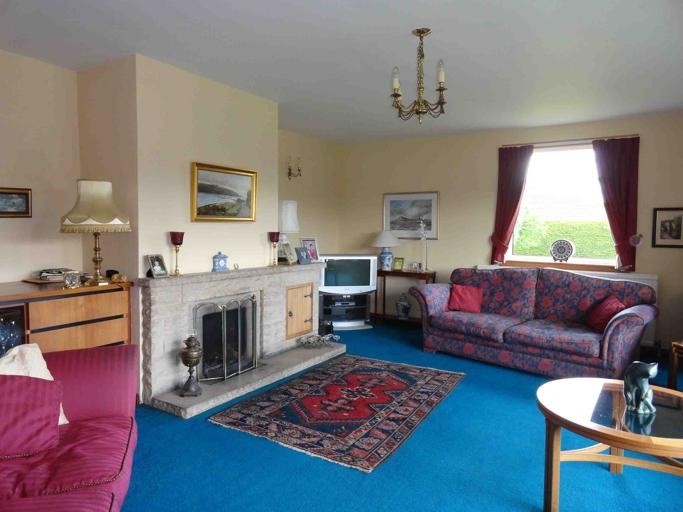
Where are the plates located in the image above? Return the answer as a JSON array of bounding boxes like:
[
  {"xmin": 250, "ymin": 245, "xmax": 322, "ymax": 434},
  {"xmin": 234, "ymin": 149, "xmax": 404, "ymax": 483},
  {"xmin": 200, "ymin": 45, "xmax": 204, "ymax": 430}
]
[{"xmin": 551, "ymin": 239, "xmax": 572, "ymax": 260}]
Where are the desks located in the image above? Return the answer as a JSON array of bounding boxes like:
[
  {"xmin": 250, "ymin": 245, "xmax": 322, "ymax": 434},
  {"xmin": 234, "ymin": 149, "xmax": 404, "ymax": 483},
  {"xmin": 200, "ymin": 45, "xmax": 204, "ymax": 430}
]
[{"xmin": 374, "ymin": 267, "xmax": 436, "ymax": 324}]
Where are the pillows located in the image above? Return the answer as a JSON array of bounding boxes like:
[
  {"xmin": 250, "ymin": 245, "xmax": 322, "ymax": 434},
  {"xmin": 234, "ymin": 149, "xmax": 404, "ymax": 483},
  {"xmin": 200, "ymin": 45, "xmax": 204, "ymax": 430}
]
[
  {"xmin": 448, "ymin": 281, "xmax": 484, "ymax": 313},
  {"xmin": 582, "ymin": 294, "xmax": 625, "ymax": 334},
  {"xmin": 1, "ymin": 345, "xmax": 69, "ymax": 462}
]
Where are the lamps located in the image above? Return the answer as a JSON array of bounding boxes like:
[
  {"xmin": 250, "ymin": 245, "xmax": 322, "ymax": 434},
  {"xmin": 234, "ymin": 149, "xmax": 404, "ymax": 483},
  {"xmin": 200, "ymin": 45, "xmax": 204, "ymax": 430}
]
[
  {"xmin": 169, "ymin": 232, "xmax": 186, "ymax": 278},
  {"xmin": 58, "ymin": 180, "xmax": 133, "ymax": 287},
  {"xmin": 269, "ymin": 232, "xmax": 282, "ymax": 268},
  {"xmin": 368, "ymin": 231, "xmax": 402, "ymax": 271},
  {"xmin": 390, "ymin": 27, "xmax": 448, "ymax": 125}
]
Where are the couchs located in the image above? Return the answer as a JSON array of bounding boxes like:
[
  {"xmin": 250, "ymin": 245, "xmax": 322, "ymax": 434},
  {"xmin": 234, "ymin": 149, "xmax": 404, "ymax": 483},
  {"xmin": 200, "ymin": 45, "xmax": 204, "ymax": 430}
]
[{"xmin": 409, "ymin": 267, "xmax": 661, "ymax": 380}]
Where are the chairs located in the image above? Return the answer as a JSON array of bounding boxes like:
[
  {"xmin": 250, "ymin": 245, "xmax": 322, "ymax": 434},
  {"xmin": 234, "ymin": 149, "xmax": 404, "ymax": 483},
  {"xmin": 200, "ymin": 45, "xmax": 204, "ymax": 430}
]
[{"xmin": 1, "ymin": 344, "xmax": 141, "ymax": 512}]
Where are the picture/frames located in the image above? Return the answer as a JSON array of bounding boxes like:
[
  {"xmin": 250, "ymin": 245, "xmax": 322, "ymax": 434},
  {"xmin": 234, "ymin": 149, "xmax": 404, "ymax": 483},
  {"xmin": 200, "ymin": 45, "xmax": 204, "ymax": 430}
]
[
  {"xmin": 652, "ymin": 207, "xmax": 683, "ymax": 248},
  {"xmin": 383, "ymin": 190, "xmax": 440, "ymax": 241},
  {"xmin": 0, "ymin": 187, "xmax": 33, "ymax": 218},
  {"xmin": 146, "ymin": 253, "xmax": 169, "ymax": 279},
  {"xmin": 282, "ymin": 237, "xmax": 322, "ymax": 266},
  {"xmin": 190, "ymin": 160, "xmax": 258, "ymax": 222}
]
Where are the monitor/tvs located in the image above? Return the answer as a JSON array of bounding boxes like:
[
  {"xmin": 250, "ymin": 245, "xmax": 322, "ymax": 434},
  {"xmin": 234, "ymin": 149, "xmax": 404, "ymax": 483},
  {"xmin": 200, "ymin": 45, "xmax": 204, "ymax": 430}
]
[{"xmin": 318, "ymin": 256, "xmax": 377, "ymax": 298}]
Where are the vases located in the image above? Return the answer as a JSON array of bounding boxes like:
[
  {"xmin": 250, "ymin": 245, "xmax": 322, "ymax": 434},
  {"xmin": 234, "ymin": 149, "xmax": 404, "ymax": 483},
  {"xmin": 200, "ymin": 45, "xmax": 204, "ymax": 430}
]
[{"xmin": 395, "ymin": 294, "xmax": 411, "ymax": 321}]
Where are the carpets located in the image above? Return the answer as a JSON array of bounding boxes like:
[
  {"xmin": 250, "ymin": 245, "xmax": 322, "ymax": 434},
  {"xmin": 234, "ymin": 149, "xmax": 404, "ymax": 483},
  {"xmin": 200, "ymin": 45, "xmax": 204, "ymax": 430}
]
[{"xmin": 206, "ymin": 351, "xmax": 468, "ymax": 475}]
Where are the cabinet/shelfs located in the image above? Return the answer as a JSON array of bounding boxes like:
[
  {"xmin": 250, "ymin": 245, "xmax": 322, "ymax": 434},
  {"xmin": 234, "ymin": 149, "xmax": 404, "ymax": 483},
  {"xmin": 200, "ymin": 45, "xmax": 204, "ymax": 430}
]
[
  {"xmin": 0, "ymin": 271, "xmax": 134, "ymax": 352},
  {"xmin": 318, "ymin": 290, "xmax": 376, "ymax": 328}
]
